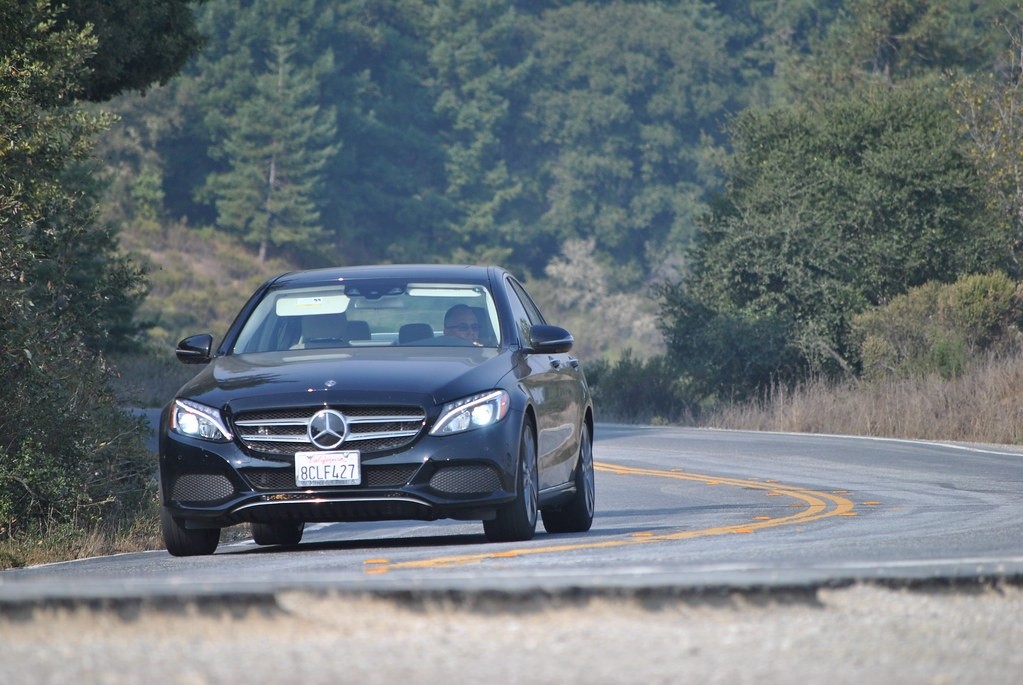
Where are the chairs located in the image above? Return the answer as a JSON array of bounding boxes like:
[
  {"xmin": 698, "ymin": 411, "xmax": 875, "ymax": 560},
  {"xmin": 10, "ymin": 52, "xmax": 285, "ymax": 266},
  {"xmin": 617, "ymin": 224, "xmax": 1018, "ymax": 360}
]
[
  {"xmin": 398, "ymin": 323, "xmax": 434, "ymax": 343},
  {"xmin": 472, "ymin": 307, "xmax": 497, "ymax": 345},
  {"xmin": 347, "ymin": 321, "xmax": 371, "ymax": 340},
  {"xmin": 301, "ymin": 312, "xmax": 352, "ymax": 347}
]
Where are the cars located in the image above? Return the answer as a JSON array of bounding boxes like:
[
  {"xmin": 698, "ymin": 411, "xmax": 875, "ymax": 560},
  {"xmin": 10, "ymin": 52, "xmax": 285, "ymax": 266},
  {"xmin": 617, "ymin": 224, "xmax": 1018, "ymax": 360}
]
[{"xmin": 155, "ymin": 262, "xmax": 595, "ymax": 558}]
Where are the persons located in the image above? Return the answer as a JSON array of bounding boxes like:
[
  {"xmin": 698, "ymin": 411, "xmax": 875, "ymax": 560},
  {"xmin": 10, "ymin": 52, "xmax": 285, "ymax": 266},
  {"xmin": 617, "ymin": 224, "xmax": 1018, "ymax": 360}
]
[{"xmin": 444, "ymin": 304, "xmax": 479, "ymax": 344}]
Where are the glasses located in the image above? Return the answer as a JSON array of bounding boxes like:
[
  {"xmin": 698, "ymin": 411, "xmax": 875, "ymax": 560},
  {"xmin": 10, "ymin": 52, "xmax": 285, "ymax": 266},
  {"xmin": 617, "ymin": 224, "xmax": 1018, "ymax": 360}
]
[{"xmin": 446, "ymin": 323, "xmax": 480, "ymax": 332}]
